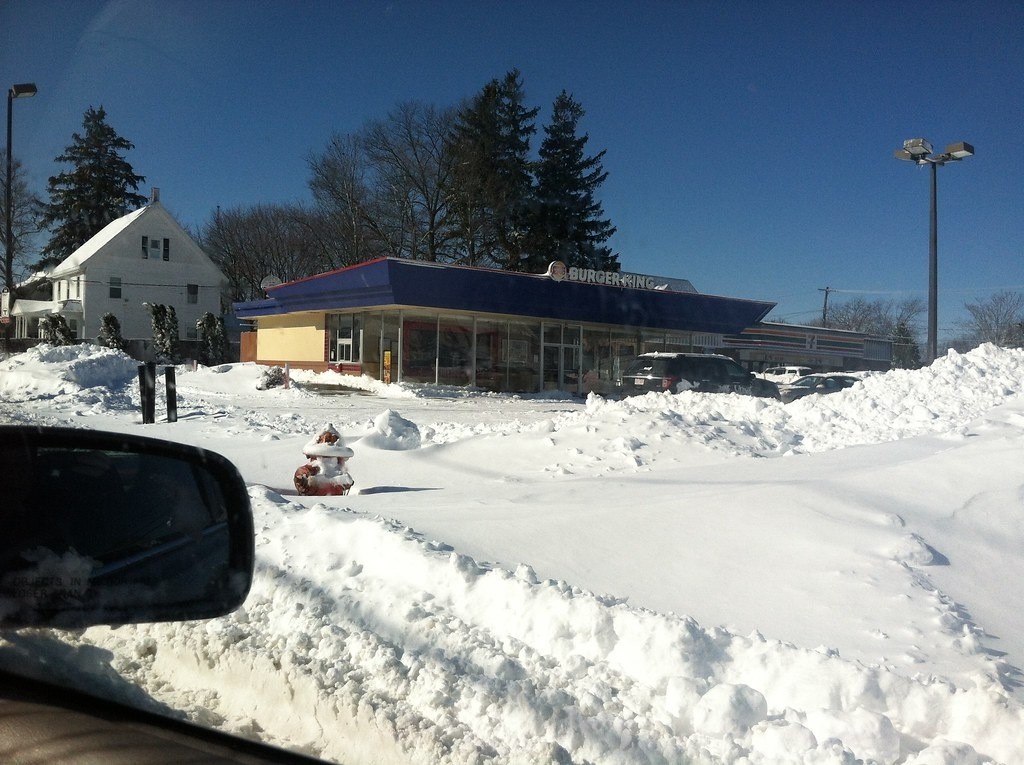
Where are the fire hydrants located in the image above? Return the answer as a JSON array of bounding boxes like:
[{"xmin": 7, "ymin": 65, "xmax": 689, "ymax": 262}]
[{"xmin": 293, "ymin": 424, "xmax": 355, "ymax": 497}]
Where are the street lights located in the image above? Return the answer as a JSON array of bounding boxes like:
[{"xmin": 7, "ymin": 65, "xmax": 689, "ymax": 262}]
[
  {"xmin": 892, "ymin": 138, "xmax": 975, "ymax": 367},
  {"xmin": 6, "ymin": 83, "xmax": 38, "ymax": 290}
]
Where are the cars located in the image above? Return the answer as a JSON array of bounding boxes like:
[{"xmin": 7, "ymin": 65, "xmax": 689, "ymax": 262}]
[
  {"xmin": 780, "ymin": 374, "xmax": 863, "ymax": 405},
  {"xmin": 760, "ymin": 367, "xmax": 813, "ymax": 386},
  {"xmin": 612, "ymin": 350, "xmax": 781, "ymax": 402}
]
